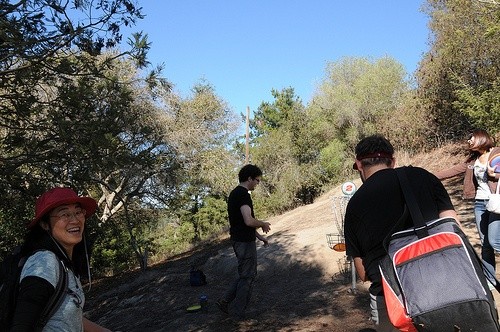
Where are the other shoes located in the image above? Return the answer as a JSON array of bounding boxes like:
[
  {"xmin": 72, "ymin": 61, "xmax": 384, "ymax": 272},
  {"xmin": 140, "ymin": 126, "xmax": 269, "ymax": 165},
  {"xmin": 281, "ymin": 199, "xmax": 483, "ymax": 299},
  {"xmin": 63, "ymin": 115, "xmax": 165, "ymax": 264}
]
[
  {"xmin": 232, "ymin": 319, "xmax": 258, "ymax": 326},
  {"xmin": 215, "ymin": 297, "xmax": 229, "ymax": 313}
]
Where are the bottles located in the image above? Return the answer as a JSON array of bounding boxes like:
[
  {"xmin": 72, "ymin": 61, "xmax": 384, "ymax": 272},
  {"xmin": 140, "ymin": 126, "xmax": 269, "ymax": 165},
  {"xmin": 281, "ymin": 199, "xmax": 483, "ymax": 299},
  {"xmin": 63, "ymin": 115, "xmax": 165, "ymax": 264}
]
[{"xmin": 200, "ymin": 296, "xmax": 208, "ymax": 314}]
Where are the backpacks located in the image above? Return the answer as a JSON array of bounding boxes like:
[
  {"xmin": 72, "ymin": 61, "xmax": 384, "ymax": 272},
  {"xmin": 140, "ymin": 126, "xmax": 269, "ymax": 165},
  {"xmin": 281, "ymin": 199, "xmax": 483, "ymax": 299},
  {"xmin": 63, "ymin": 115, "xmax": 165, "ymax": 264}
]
[{"xmin": 0, "ymin": 252, "xmax": 70, "ymax": 332}]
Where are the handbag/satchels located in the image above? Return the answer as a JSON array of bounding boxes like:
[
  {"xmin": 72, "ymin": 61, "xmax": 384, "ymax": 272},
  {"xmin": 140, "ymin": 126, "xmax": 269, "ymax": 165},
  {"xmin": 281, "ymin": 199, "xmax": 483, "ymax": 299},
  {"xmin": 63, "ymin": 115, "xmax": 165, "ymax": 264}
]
[
  {"xmin": 485, "ymin": 194, "xmax": 499, "ymax": 214},
  {"xmin": 378, "ymin": 217, "xmax": 500, "ymax": 332},
  {"xmin": 189, "ymin": 262, "xmax": 207, "ymax": 286}
]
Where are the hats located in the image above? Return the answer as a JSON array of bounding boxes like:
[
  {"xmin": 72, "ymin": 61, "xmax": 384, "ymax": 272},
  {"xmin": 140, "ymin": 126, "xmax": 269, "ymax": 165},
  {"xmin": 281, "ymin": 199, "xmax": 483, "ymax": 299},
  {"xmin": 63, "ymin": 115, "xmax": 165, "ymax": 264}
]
[
  {"xmin": 353, "ymin": 152, "xmax": 393, "ymax": 170},
  {"xmin": 24, "ymin": 187, "xmax": 97, "ymax": 231}
]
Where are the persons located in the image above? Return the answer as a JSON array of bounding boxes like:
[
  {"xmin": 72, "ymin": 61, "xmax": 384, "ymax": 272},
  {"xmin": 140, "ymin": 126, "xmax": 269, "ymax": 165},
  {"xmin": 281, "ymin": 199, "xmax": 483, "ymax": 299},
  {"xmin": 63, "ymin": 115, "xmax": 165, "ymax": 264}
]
[
  {"xmin": 343, "ymin": 133, "xmax": 464, "ymax": 332},
  {"xmin": 433, "ymin": 128, "xmax": 500, "ymax": 291},
  {"xmin": 11, "ymin": 187, "xmax": 111, "ymax": 332},
  {"xmin": 215, "ymin": 164, "xmax": 271, "ymax": 326}
]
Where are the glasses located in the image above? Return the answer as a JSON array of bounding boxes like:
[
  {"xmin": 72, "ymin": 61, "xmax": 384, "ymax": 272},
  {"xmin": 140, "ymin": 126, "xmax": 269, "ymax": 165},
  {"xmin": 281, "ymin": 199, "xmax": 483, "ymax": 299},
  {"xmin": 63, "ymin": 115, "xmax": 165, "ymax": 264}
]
[
  {"xmin": 254, "ymin": 177, "xmax": 261, "ymax": 183},
  {"xmin": 48, "ymin": 209, "xmax": 87, "ymax": 220}
]
